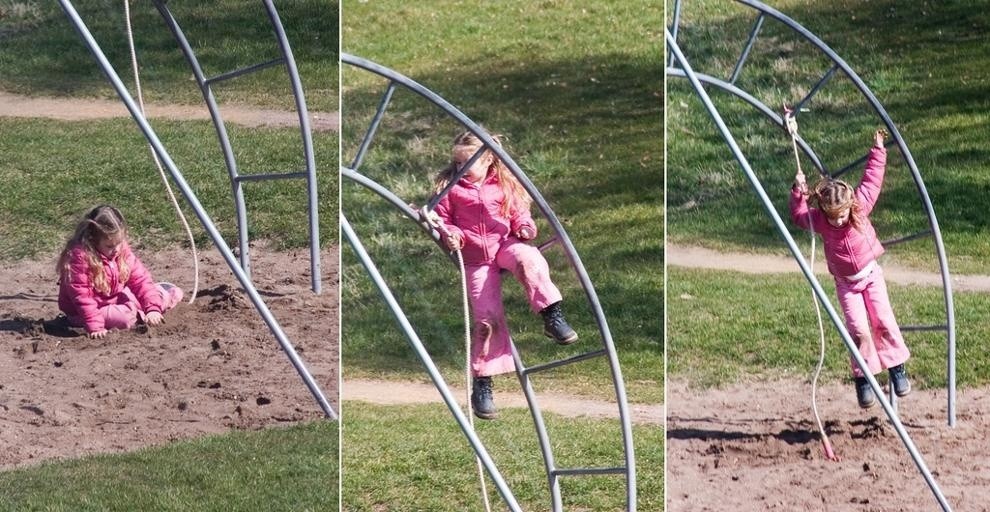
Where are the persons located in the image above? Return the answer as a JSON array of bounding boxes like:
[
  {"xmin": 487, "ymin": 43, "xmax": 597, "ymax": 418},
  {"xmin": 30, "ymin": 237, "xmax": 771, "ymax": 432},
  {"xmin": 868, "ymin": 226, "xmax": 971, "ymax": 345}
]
[
  {"xmin": 53, "ymin": 202, "xmax": 186, "ymax": 343},
  {"xmin": 425, "ymin": 128, "xmax": 579, "ymax": 423},
  {"xmin": 786, "ymin": 123, "xmax": 913, "ymax": 409}
]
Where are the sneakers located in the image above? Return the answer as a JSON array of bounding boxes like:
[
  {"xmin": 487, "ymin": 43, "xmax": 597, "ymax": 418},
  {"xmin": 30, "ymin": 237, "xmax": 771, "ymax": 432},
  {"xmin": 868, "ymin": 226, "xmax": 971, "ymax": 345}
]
[
  {"xmin": 853, "ymin": 376, "xmax": 876, "ymax": 408},
  {"xmin": 887, "ymin": 362, "xmax": 911, "ymax": 396},
  {"xmin": 540, "ymin": 302, "xmax": 579, "ymax": 345},
  {"xmin": 471, "ymin": 375, "xmax": 498, "ymax": 420}
]
